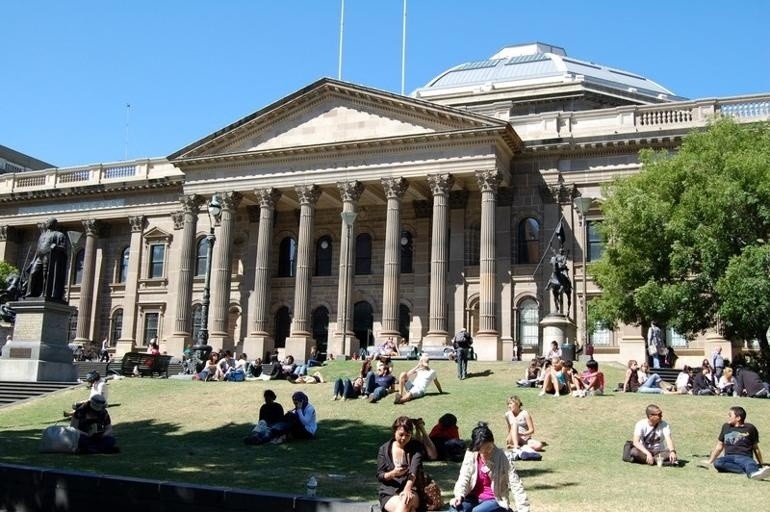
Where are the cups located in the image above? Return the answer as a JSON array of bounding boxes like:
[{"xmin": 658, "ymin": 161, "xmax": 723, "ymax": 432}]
[{"xmin": 656, "ymin": 457, "xmax": 664, "ymax": 469}]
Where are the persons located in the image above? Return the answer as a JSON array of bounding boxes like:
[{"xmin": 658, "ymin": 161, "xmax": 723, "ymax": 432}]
[
  {"xmin": 413, "ymin": 418, "xmax": 466, "ymax": 463},
  {"xmin": 550, "ymin": 247, "xmax": 571, "ymax": 289},
  {"xmin": 452, "ymin": 328, "xmax": 469, "ymax": 380},
  {"xmin": 327, "ymin": 336, "xmax": 441, "ymax": 402},
  {"xmin": 100, "ymin": 335, "xmax": 110, "ymax": 362},
  {"xmin": 429, "ymin": 412, "xmax": 460, "ymax": 440},
  {"xmin": 259, "ymin": 388, "xmax": 284, "ymax": 426},
  {"xmin": 5, "ymin": 334, "xmax": 12, "ymax": 344},
  {"xmin": 70, "ymin": 394, "xmax": 121, "ymax": 454},
  {"xmin": 24, "ymin": 216, "xmax": 67, "ymax": 297},
  {"xmin": 376, "ymin": 415, "xmax": 426, "ymax": 512},
  {"xmin": 447, "ymin": 420, "xmax": 530, "ymax": 512},
  {"xmin": 515, "ymin": 341, "xmax": 604, "ymax": 397},
  {"xmin": 63, "ymin": 370, "xmax": 108, "ymax": 417},
  {"xmin": 131, "ymin": 334, "xmax": 159, "ymax": 377},
  {"xmin": 270, "ymin": 390, "xmax": 318, "ymax": 445},
  {"xmin": 620, "ymin": 346, "xmax": 770, "ymax": 399},
  {"xmin": 704, "ymin": 407, "xmax": 770, "ymax": 481},
  {"xmin": 630, "ymin": 403, "xmax": 678, "ymax": 466},
  {"xmin": 504, "ymin": 395, "xmax": 534, "ymax": 449},
  {"xmin": 648, "ymin": 320, "xmax": 665, "ymax": 368},
  {"xmin": 184, "ymin": 344, "xmax": 324, "ymax": 385}
]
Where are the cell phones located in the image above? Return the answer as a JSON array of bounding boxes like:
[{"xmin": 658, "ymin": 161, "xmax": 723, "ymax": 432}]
[{"xmin": 401, "ymin": 464, "xmax": 408, "ymax": 471}]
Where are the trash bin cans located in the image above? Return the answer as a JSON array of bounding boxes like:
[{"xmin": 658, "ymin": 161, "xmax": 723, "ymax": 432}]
[{"xmin": 561, "ymin": 343, "xmax": 576, "ymax": 361}]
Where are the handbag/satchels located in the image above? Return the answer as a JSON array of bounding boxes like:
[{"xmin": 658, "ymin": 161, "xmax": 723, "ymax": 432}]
[
  {"xmin": 623, "ymin": 441, "xmax": 634, "ymax": 462},
  {"xmin": 39, "ymin": 423, "xmax": 81, "ymax": 453},
  {"xmin": 424, "ymin": 479, "xmax": 442, "ymax": 511},
  {"xmin": 647, "ymin": 345, "xmax": 657, "ymax": 356}
]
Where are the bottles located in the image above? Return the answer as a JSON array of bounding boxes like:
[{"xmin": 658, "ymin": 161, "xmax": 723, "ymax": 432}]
[{"xmin": 306, "ymin": 475, "xmax": 317, "ymax": 497}]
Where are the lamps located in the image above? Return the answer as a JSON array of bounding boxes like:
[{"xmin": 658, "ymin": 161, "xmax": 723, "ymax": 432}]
[{"xmin": 123, "ymin": 251, "xmax": 130, "ymax": 259}]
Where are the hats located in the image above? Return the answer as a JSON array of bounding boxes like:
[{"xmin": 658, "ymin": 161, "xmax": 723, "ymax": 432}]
[{"xmin": 91, "ymin": 394, "xmax": 106, "ymax": 406}]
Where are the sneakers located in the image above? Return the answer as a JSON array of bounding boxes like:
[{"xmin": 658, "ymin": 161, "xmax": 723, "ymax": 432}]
[{"xmin": 751, "ymin": 466, "xmax": 770, "ymax": 480}]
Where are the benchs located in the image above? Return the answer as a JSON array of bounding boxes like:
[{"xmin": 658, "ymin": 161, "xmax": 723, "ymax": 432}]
[
  {"xmin": 109, "ymin": 353, "xmax": 174, "ymax": 379},
  {"xmin": 365, "ymin": 346, "xmax": 418, "ymax": 361},
  {"xmin": 421, "ymin": 346, "xmax": 453, "ymax": 361}
]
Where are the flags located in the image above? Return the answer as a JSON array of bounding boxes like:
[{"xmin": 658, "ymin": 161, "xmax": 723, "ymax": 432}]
[{"xmin": 555, "ymin": 223, "xmax": 566, "ymax": 243}]
